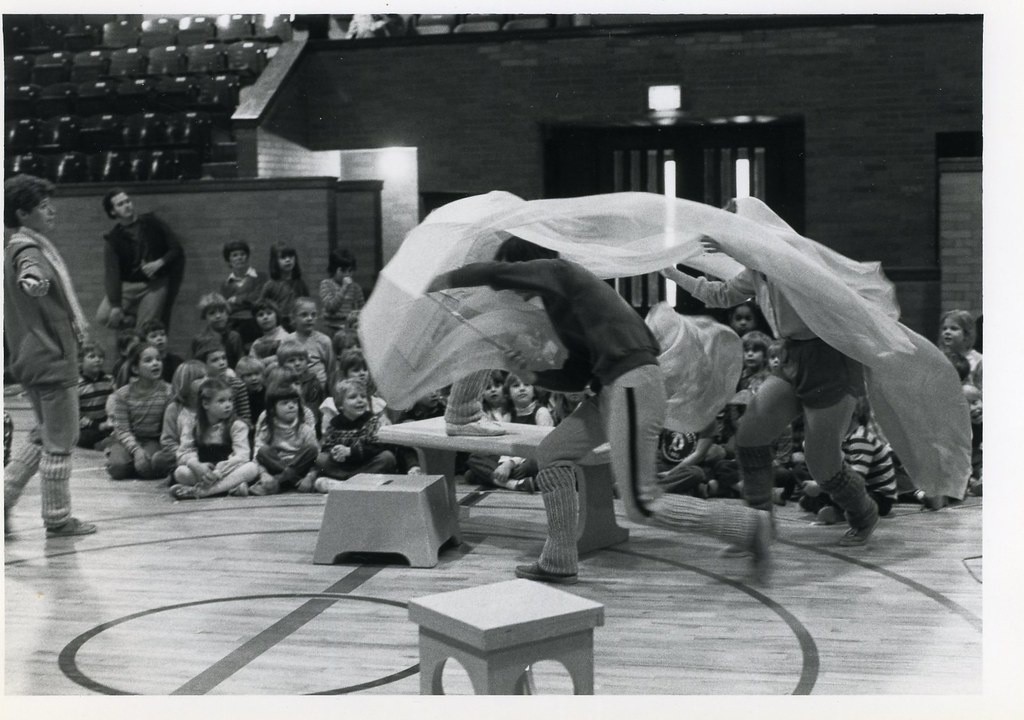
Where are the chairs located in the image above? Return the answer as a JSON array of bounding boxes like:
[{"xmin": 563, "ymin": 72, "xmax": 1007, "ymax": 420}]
[{"xmin": 3, "ymin": 14, "xmax": 554, "ymax": 185}]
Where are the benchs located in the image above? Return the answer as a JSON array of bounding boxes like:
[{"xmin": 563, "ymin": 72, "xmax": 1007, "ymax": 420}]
[{"xmin": 377, "ymin": 415, "xmax": 630, "ymax": 556}]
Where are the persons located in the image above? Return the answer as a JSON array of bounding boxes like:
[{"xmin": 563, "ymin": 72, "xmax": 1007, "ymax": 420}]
[
  {"xmin": 96, "ymin": 187, "xmax": 185, "ymax": 330},
  {"xmin": 220, "ymin": 239, "xmax": 268, "ymax": 343},
  {"xmin": 192, "ymin": 293, "xmax": 241, "ymax": 369},
  {"xmin": 249, "ymin": 302, "xmax": 289, "ymax": 374},
  {"xmin": 316, "ymin": 376, "xmax": 383, "ymax": 481},
  {"xmin": 4, "ymin": 172, "xmax": 97, "ymax": 536},
  {"xmin": 612, "ymin": 303, "xmax": 983, "ymax": 523},
  {"xmin": 268, "ymin": 337, "xmax": 323, "ymax": 422},
  {"xmin": 249, "ymin": 381, "xmax": 320, "ymax": 494},
  {"xmin": 660, "ymin": 197, "xmax": 881, "ymax": 547},
  {"xmin": 319, "ymin": 249, "xmax": 393, "ymax": 433},
  {"xmin": 426, "ymin": 236, "xmax": 772, "ymax": 584},
  {"xmin": 79, "ymin": 343, "xmax": 117, "ymax": 449},
  {"xmin": 236, "ymin": 356, "xmax": 266, "ymax": 423},
  {"xmin": 106, "ymin": 320, "xmax": 265, "ymax": 499},
  {"xmin": 390, "ymin": 367, "xmax": 588, "ymax": 494},
  {"xmin": 289, "ymin": 296, "xmax": 335, "ymax": 398},
  {"xmin": 263, "ymin": 239, "xmax": 310, "ymax": 334}
]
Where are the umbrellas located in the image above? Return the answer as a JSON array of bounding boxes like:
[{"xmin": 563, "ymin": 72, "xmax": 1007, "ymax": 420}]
[{"xmin": 358, "ymin": 190, "xmax": 527, "ymax": 390}]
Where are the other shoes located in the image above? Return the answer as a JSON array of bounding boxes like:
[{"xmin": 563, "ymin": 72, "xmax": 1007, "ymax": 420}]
[
  {"xmin": 970, "ymin": 482, "xmax": 982, "ymax": 496},
  {"xmin": 836, "ymin": 515, "xmax": 882, "ymax": 544},
  {"xmin": 315, "ymin": 477, "xmax": 339, "ymax": 492},
  {"xmin": 445, "ymin": 415, "xmax": 507, "ymax": 436},
  {"xmin": 229, "ymin": 481, "xmax": 248, "ymax": 496},
  {"xmin": 799, "ymin": 496, "xmax": 808, "ymax": 508},
  {"xmin": 45, "ymin": 518, "xmax": 96, "ymax": 536},
  {"xmin": 772, "ymin": 487, "xmax": 786, "ymax": 506},
  {"xmin": 515, "ymin": 561, "xmax": 578, "ymax": 584},
  {"xmin": 696, "ymin": 483, "xmax": 709, "ymax": 498},
  {"xmin": 250, "ymin": 477, "xmax": 282, "ymax": 496},
  {"xmin": 517, "ymin": 476, "xmax": 534, "ymax": 493},
  {"xmin": 818, "ymin": 506, "xmax": 841, "ymax": 523},
  {"xmin": 923, "ymin": 492, "xmax": 950, "ymax": 508},
  {"xmin": 464, "ymin": 469, "xmax": 482, "ymax": 484},
  {"xmin": 169, "ymin": 484, "xmax": 200, "ymax": 499}
]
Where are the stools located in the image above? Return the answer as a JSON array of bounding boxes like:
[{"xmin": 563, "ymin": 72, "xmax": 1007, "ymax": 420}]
[
  {"xmin": 408, "ymin": 578, "xmax": 604, "ymax": 695},
  {"xmin": 313, "ymin": 473, "xmax": 462, "ymax": 568}
]
